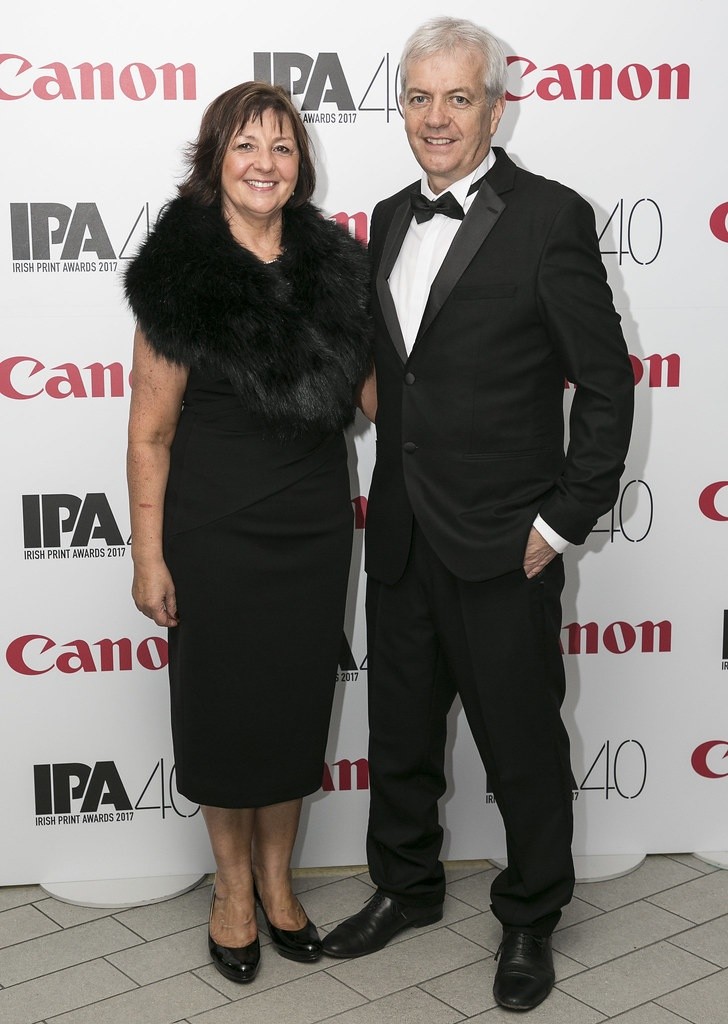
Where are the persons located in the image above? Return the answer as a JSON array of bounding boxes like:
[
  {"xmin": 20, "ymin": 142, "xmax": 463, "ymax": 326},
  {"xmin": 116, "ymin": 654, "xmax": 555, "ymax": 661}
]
[
  {"xmin": 321, "ymin": 18, "xmax": 635, "ymax": 1013},
  {"xmin": 127, "ymin": 83, "xmax": 378, "ymax": 988}
]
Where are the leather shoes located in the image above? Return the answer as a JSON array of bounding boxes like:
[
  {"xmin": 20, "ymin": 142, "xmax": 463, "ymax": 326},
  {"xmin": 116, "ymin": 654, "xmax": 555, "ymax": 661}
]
[
  {"xmin": 493, "ymin": 925, "xmax": 555, "ymax": 1010},
  {"xmin": 321, "ymin": 892, "xmax": 443, "ymax": 958},
  {"xmin": 254, "ymin": 877, "xmax": 323, "ymax": 962},
  {"xmin": 207, "ymin": 879, "xmax": 261, "ymax": 983}
]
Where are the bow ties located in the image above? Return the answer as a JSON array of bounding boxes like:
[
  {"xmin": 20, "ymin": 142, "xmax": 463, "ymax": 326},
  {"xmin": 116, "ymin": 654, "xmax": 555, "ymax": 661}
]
[{"xmin": 407, "ymin": 169, "xmax": 489, "ymax": 225}]
